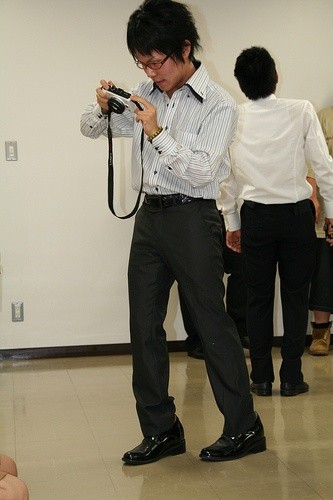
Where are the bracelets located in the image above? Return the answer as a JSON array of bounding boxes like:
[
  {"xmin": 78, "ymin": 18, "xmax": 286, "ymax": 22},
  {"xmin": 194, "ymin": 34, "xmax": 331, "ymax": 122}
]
[{"xmin": 147, "ymin": 127, "xmax": 163, "ymax": 141}]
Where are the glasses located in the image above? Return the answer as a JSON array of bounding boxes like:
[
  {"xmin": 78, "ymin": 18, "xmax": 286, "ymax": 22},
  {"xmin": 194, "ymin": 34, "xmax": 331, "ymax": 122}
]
[{"xmin": 134, "ymin": 52, "xmax": 172, "ymax": 69}]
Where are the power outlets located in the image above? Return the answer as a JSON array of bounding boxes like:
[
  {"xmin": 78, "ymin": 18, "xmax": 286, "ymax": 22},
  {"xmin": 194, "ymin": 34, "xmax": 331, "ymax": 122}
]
[
  {"xmin": 12, "ymin": 303, "xmax": 23, "ymax": 321},
  {"xmin": 5, "ymin": 141, "xmax": 18, "ymax": 160}
]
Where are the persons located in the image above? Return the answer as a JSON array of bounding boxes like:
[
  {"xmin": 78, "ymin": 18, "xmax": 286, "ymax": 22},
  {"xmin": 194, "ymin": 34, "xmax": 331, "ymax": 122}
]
[
  {"xmin": 216, "ymin": 46, "xmax": 333, "ymax": 396},
  {"xmin": 306, "ymin": 105, "xmax": 333, "ymax": 356},
  {"xmin": 80, "ymin": 0, "xmax": 267, "ymax": 465},
  {"xmin": 176, "ymin": 211, "xmax": 251, "ymax": 361}
]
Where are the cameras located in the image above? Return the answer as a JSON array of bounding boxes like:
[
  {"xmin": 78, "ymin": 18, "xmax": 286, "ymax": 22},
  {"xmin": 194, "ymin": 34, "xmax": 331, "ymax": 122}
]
[{"xmin": 101, "ymin": 85, "xmax": 144, "ymax": 114}]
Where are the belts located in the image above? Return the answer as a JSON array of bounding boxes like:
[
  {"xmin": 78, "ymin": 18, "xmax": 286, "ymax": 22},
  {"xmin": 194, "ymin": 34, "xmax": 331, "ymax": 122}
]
[{"xmin": 144, "ymin": 193, "xmax": 194, "ymax": 207}]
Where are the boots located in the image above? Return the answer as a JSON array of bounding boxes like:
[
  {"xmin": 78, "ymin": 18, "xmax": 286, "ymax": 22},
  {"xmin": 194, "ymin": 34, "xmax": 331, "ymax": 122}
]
[{"xmin": 310, "ymin": 321, "xmax": 332, "ymax": 355}]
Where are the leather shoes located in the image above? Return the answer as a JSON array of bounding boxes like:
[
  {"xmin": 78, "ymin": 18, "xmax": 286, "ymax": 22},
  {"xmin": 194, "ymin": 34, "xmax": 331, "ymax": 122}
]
[
  {"xmin": 249, "ymin": 382, "xmax": 272, "ymax": 397},
  {"xmin": 280, "ymin": 382, "xmax": 309, "ymax": 396},
  {"xmin": 199, "ymin": 411, "xmax": 267, "ymax": 461},
  {"xmin": 187, "ymin": 350, "xmax": 205, "ymax": 359},
  {"xmin": 122, "ymin": 414, "xmax": 186, "ymax": 464}
]
